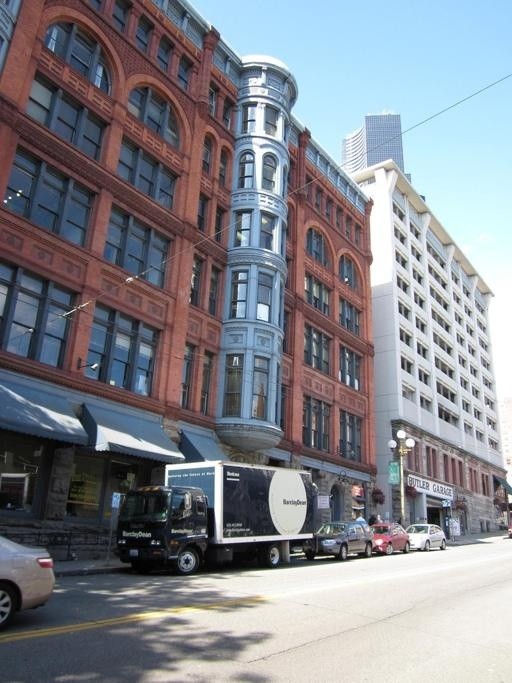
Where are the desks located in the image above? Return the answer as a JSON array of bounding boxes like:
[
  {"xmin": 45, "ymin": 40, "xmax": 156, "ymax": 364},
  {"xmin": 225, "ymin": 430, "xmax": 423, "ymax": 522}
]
[{"xmin": 386, "ymin": 428, "xmax": 415, "ymax": 521}]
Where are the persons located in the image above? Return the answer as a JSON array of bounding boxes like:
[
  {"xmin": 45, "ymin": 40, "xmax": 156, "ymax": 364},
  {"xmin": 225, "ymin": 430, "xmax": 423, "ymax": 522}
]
[{"xmin": 367, "ymin": 514, "xmax": 384, "ymax": 526}]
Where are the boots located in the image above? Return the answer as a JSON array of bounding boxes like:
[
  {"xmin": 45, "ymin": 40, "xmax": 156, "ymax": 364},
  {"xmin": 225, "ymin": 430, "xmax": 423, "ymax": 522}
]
[{"xmin": 302, "ymin": 520, "xmax": 373, "ymax": 561}]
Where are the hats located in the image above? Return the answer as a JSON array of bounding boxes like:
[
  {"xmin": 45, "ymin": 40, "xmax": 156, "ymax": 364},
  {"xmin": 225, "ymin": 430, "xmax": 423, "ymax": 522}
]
[
  {"xmin": 404, "ymin": 523, "xmax": 447, "ymax": 552},
  {"xmin": 369, "ymin": 522, "xmax": 411, "ymax": 554},
  {"xmin": 0, "ymin": 529, "xmax": 55, "ymax": 629}
]
